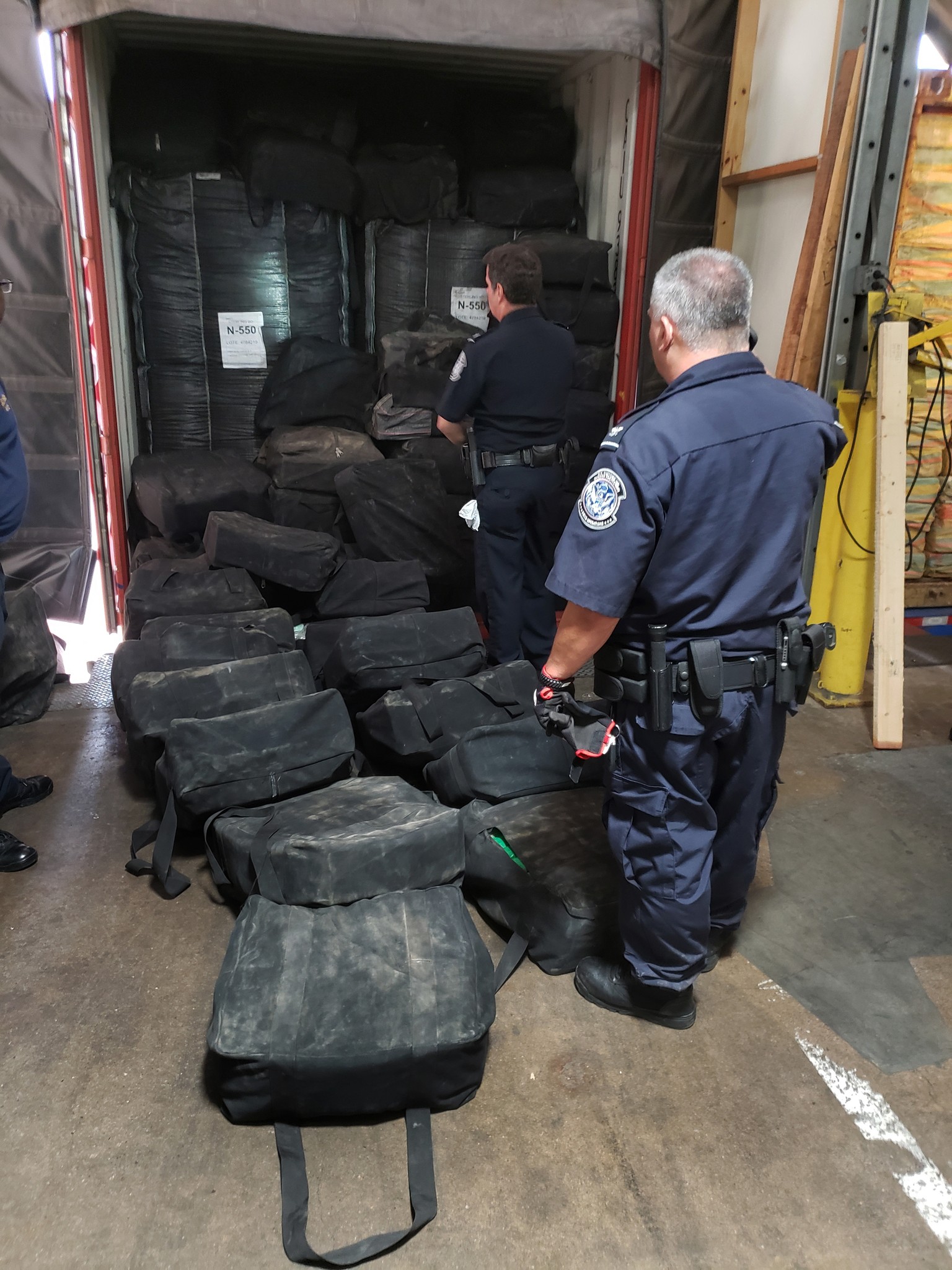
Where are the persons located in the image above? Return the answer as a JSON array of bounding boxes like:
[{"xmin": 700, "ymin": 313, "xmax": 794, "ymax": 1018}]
[
  {"xmin": 436, "ymin": 242, "xmax": 580, "ymax": 698},
  {"xmin": 534, "ymin": 246, "xmax": 848, "ymax": 1029},
  {"xmin": 0, "ymin": 280, "xmax": 54, "ymax": 872}
]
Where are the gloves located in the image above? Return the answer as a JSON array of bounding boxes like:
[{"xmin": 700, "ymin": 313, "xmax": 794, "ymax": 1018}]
[{"xmin": 533, "ymin": 668, "xmax": 621, "ymax": 761}]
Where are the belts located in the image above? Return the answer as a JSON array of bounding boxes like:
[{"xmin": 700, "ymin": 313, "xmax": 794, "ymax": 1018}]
[
  {"xmin": 672, "ymin": 654, "xmax": 775, "ymax": 692},
  {"xmin": 492, "ymin": 453, "xmax": 522, "ymax": 466}
]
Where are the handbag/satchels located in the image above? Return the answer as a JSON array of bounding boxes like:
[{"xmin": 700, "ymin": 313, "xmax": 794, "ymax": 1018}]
[
  {"xmin": 357, "ymin": 142, "xmax": 459, "ymax": 223},
  {"xmin": 468, "ymin": 164, "xmax": 586, "ymax": 228},
  {"xmin": 515, "ymin": 232, "xmax": 611, "ymax": 327},
  {"xmin": 247, "ymin": 136, "xmax": 358, "ymax": 220},
  {"xmin": 102, "ymin": 330, "xmax": 618, "ymax": 1270},
  {"xmin": 469, "ymin": 104, "xmax": 574, "ymax": 164},
  {"xmin": 535, "ymin": 283, "xmax": 620, "ymax": 344},
  {"xmin": 0, "ymin": 573, "xmax": 57, "ymax": 729},
  {"xmin": 124, "ymin": 116, "xmax": 220, "ymax": 177}
]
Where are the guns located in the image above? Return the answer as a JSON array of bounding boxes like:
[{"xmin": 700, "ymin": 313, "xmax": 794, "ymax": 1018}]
[{"xmin": 797, "ymin": 619, "xmax": 838, "ymax": 707}]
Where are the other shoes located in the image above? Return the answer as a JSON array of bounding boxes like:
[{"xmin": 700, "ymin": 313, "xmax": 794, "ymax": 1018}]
[
  {"xmin": 575, "ymin": 956, "xmax": 696, "ymax": 1030},
  {"xmin": 0, "ymin": 829, "xmax": 37, "ymax": 873},
  {"xmin": 0, "ymin": 774, "xmax": 54, "ymax": 819},
  {"xmin": 700, "ymin": 933, "xmax": 734, "ymax": 973}
]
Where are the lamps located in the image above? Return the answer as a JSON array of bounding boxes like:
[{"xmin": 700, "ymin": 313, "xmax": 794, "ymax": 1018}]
[{"xmin": 919, "ymin": 28, "xmax": 950, "ymax": 71}]
